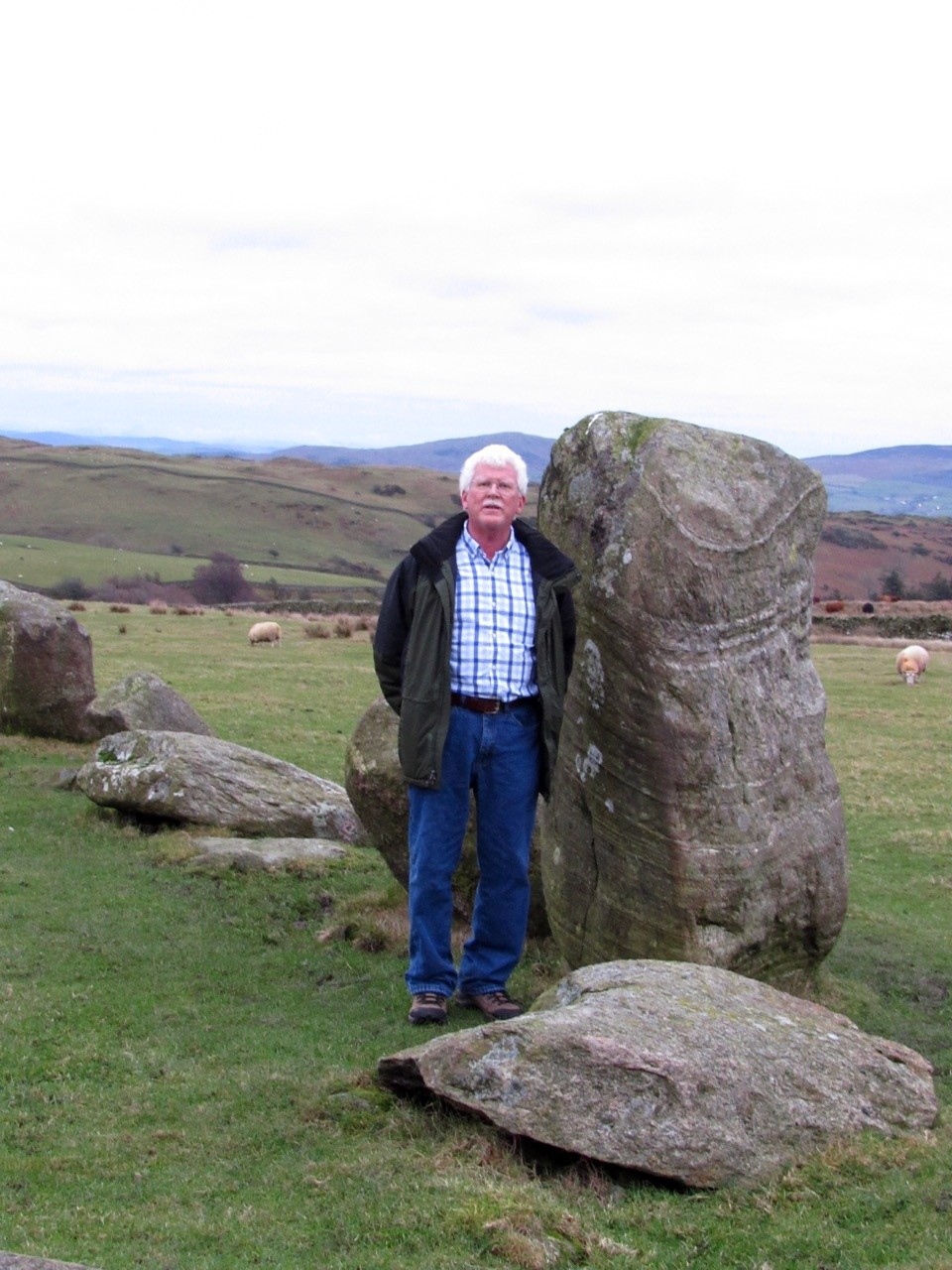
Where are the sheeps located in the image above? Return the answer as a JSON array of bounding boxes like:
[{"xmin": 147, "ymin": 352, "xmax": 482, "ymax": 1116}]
[
  {"xmin": 896, "ymin": 645, "xmax": 930, "ymax": 677},
  {"xmin": 249, "ymin": 621, "xmax": 281, "ymax": 646}
]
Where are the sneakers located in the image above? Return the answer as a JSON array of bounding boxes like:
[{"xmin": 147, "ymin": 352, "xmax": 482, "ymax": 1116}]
[
  {"xmin": 408, "ymin": 990, "xmax": 448, "ymax": 1026},
  {"xmin": 454, "ymin": 986, "xmax": 524, "ymax": 1021}
]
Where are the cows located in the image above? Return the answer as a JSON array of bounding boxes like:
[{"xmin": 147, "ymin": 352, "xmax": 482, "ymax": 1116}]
[
  {"xmin": 862, "ymin": 603, "xmax": 874, "ymax": 613},
  {"xmin": 878, "ymin": 593, "xmax": 901, "ymax": 602},
  {"xmin": 825, "ymin": 602, "xmax": 844, "ymax": 612}
]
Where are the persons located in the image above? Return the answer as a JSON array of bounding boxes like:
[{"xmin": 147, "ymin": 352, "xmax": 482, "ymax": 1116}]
[{"xmin": 371, "ymin": 444, "xmax": 583, "ymax": 1026}]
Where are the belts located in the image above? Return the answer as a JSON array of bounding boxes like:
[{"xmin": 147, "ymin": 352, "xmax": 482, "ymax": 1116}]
[{"xmin": 450, "ymin": 692, "xmax": 538, "ymax": 715}]
